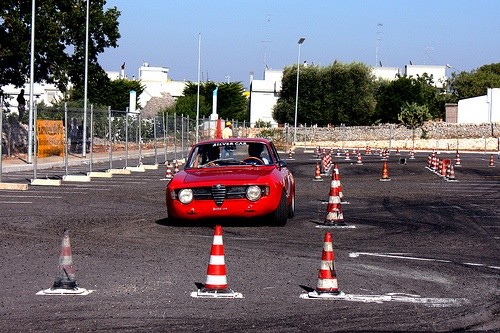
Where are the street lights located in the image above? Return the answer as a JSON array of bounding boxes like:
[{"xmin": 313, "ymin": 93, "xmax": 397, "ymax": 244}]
[{"xmin": 294, "ymin": 38, "xmax": 305, "ymax": 146}]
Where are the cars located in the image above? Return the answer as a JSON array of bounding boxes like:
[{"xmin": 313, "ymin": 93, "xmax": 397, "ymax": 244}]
[{"xmin": 165, "ymin": 138, "xmax": 295, "ymax": 227}]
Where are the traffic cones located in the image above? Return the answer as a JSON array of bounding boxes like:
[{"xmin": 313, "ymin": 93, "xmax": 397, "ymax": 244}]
[
  {"xmin": 488, "ymin": 155, "xmax": 495, "ymax": 167},
  {"xmin": 426, "ymin": 151, "xmax": 446, "ymax": 176},
  {"xmin": 44, "ymin": 228, "xmax": 86, "ymax": 294},
  {"xmin": 308, "ymin": 232, "xmax": 346, "ymax": 298},
  {"xmin": 174, "ymin": 159, "xmax": 180, "ymax": 175},
  {"xmin": 380, "ymin": 161, "xmax": 391, "ymax": 181},
  {"xmin": 322, "ymin": 163, "xmax": 348, "ymax": 203},
  {"xmin": 315, "ymin": 147, "xmax": 415, "ymax": 174},
  {"xmin": 455, "ymin": 154, "xmax": 461, "ymax": 165},
  {"xmin": 288, "ymin": 149, "xmax": 292, "ymax": 159},
  {"xmin": 449, "ymin": 165, "xmax": 457, "ymax": 181},
  {"xmin": 165, "ymin": 163, "xmax": 173, "ymax": 179},
  {"xmin": 196, "ymin": 226, "xmax": 239, "ymax": 297},
  {"xmin": 446, "ymin": 165, "xmax": 450, "ymax": 178},
  {"xmin": 319, "ymin": 171, "xmax": 348, "ymax": 226},
  {"xmin": 313, "ymin": 162, "xmax": 324, "ymax": 181}
]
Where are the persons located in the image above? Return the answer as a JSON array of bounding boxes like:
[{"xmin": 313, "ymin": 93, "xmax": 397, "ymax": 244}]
[
  {"xmin": 242, "ymin": 143, "xmax": 269, "ymax": 165},
  {"xmin": 221, "ymin": 122, "xmax": 234, "ymax": 159},
  {"xmin": 17, "ymin": 89, "xmax": 26, "ymax": 123},
  {"xmin": 194, "ymin": 147, "xmax": 220, "ymax": 167},
  {"xmin": 69, "ymin": 118, "xmax": 79, "ymax": 153},
  {"xmin": 79, "ymin": 120, "xmax": 90, "ymax": 152}
]
